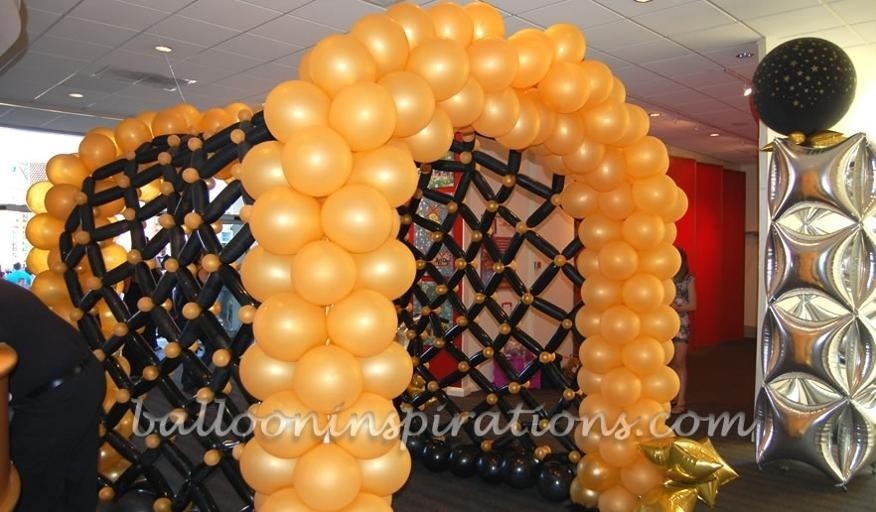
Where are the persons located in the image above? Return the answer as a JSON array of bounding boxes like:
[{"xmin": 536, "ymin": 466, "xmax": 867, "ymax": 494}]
[{"xmin": 1, "ymin": 259, "xmax": 106, "ymax": 512}]
